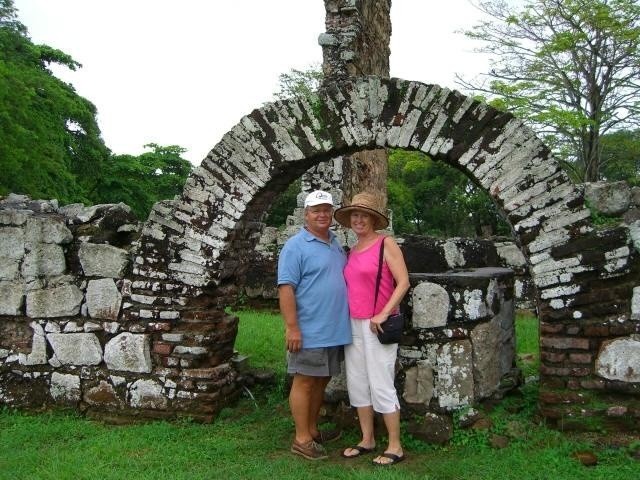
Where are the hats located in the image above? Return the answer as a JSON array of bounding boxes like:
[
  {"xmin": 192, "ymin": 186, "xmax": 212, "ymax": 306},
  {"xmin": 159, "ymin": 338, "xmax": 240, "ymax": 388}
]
[
  {"xmin": 304, "ymin": 190, "xmax": 334, "ymax": 209},
  {"xmin": 334, "ymin": 193, "xmax": 389, "ymax": 230}
]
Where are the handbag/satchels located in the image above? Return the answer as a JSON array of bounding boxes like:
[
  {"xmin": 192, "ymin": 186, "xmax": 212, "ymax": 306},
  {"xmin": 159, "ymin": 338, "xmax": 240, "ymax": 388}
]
[
  {"xmin": 402, "ymin": 241, "xmax": 449, "ymax": 272},
  {"xmin": 377, "ymin": 314, "xmax": 404, "ymax": 343}
]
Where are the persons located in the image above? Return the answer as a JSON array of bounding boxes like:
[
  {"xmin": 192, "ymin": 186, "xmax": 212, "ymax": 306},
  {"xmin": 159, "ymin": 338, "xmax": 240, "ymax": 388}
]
[
  {"xmin": 275, "ymin": 190, "xmax": 351, "ymax": 460},
  {"xmin": 331, "ymin": 194, "xmax": 413, "ymax": 466}
]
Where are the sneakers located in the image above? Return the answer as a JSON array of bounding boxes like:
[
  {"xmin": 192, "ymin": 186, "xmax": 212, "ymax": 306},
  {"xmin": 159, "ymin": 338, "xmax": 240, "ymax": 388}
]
[{"xmin": 291, "ymin": 427, "xmax": 342, "ymax": 460}]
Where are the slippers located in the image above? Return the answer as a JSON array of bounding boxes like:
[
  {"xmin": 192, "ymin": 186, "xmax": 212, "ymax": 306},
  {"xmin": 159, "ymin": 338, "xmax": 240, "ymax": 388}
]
[
  {"xmin": 372, "ymin": 449, "xmax": 406, "ymax": 466},
  {"xmin": 341, "ymin": 444, "xmax": 377, "ymax": 458}
]
